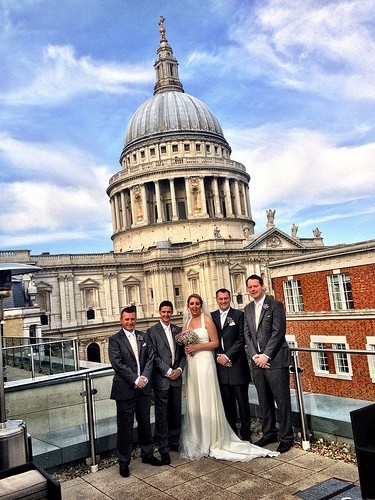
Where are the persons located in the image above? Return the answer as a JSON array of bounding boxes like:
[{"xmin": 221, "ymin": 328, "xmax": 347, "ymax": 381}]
[
  {"xmin": 108, "ymin": 307, "xmax": 162, "ymax": 477},
  {"xmin": 145, "ymin": 300, "xmax": 186, "ymax": 457},
  {"xmin": 243, "ymin": 275, "xmax": 293, "ymax": 453},
  {"xmin": 180, "ymin": 293, "xmax": 280, "ymax": 463},
  {"xmin": 210, "ymin": 289, "xmax": 253, "ymax": 444}
]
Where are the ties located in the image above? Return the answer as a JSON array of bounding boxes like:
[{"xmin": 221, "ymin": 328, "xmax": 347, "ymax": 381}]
[
  {"xmin": 129, "ymin": 333, "xmax": 140, "ymax": 377},
  {"xmin": 166, "ymin": 327, "xmax": 175, "ymax": 367},
  {"xmin": 255, "ymin": 303, "xmax": 261, "ymax": 353},
  {"xmin": 220, "ymin": 312, "xmax": 226, "ymax": 350}
]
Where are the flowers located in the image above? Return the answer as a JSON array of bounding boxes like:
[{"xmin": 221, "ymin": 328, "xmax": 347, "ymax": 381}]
[{"xmin": 176, "ymin": 330, "xmax": 198, "ymax": 357}]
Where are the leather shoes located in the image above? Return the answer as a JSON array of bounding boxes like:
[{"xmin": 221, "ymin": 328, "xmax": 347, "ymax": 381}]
[
  {"xmin": 120, "ymin": 466, "xmax": 129, "ymax": 477},
  {"xmin": 277, "ymin": 441, "xmax": 294, "ymax": 453},
  {"xmin": 162, "ymin": 453, "xmax": 171, "ymax": 465},
  {"xmin": 242, "ymin": 436, "xmax": 252, "ymax": 443},
  {"xmin": 253, "ymin": 436, "xmax": 277, "ymax": 447},
  {"xmin": 142, "ymin": 455, "xmax": 163, "ymax": 466},
  {"xmin": 169, "ymin": 444, "xmax": 180, "ymax": 452}
]
